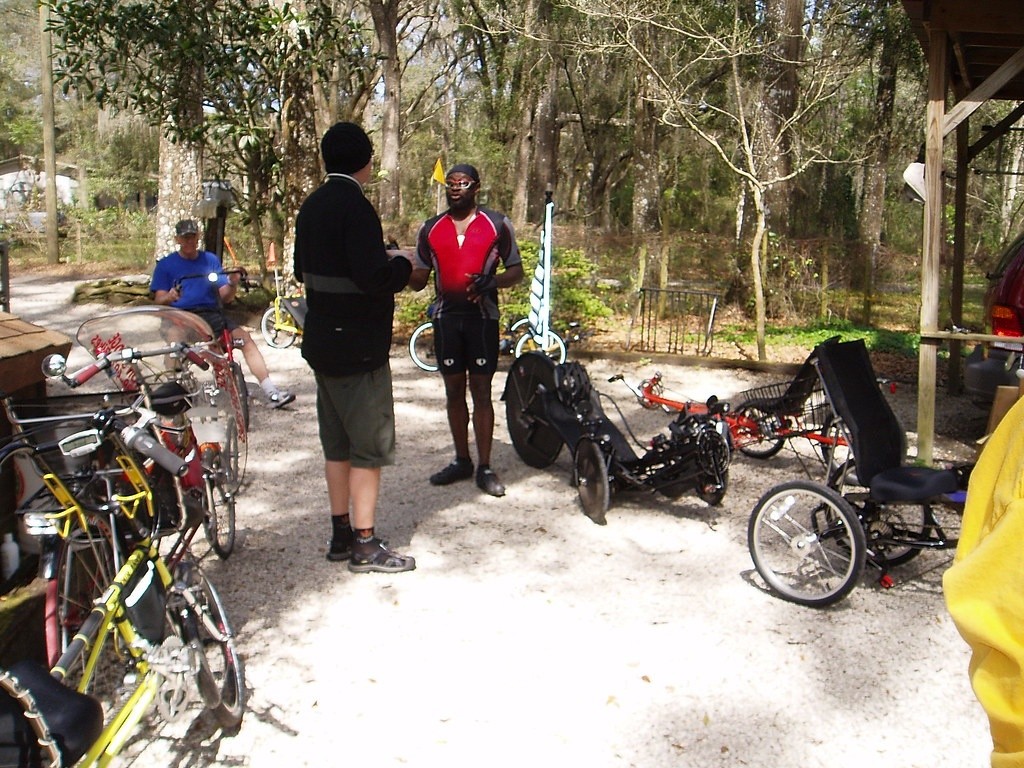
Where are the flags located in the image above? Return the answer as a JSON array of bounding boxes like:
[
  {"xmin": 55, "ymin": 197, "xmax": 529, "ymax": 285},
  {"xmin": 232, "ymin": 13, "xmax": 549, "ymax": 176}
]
[{"xmin": 267, "ymin": 242, "xmax": 276, "ymax": 267}]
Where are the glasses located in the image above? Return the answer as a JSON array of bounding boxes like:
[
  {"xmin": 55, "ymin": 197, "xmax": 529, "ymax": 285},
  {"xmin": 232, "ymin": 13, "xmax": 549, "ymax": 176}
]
[{"xmin": 444, "ymin": 179, "xmax": 476, "ymax": 189}]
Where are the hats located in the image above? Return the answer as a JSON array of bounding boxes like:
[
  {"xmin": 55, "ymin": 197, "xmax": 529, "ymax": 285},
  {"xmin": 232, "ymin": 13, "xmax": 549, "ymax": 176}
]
[
  {"xmin": 176, "ymin": 220, "xmax": 199, "ymax": 237},
  {"xmin": 321, "ymin": 121, "xmax": 373, "ymax": 176},
  {"xmin": 446, "ymin": 164, "xmax": 481, "ymax": 183}
]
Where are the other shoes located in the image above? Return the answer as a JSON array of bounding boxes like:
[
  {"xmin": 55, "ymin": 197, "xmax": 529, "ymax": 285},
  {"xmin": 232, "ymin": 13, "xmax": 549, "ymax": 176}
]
[
  {"xmin": 476, "ymin": 466, "xmax": 506, "ymax": 496},
  {"xmin": 429, "ymin": 459, "xmax": 474, "ymax": 486}
]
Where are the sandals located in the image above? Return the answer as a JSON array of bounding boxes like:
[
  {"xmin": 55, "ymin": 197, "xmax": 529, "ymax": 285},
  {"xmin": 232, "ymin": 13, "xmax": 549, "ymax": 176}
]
[
  {"xmin": 327, "ymin": 533, "xmax": 355, "ymax": 560},
  {"xmin": 348, "ymin": 543, "xmax": 416, "ymax": 571}
]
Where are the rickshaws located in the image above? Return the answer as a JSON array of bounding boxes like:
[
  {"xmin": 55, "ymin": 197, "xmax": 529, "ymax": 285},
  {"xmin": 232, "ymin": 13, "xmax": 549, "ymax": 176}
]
[
  {"xmin": 609, "ymin": 334, "xmax": 863, "ymax": 473},
  {"xmin": 500, "ymin": 347, "xmax": 733, "ymax": 522},
  {"xmin": 746, "ymin": 336, "xmax": 978, "ymax": 610},
  {"xmin": 1, "ymin": 264, "xmax": 254, "ymax": 768}
]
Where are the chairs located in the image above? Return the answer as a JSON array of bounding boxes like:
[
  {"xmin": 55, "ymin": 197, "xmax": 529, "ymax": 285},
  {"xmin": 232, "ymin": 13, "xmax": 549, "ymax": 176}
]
[{"xmin": 812, "ymin": 338, "xmax": 956, "ymax": 503}]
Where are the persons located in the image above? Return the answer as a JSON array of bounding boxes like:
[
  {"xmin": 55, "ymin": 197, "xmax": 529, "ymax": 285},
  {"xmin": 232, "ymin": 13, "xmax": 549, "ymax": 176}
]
[
  {"xmin": 405, "ymin": 164, "xmax": 525, "ymax": 496},
  {"xmin": 293, "ymin": 121, "xmax": 417, "ymax": 572},
  {"xmin": 943, "ymin": 395, "xmax": 1024, "ymax": 768},
  {"xmin": 150, "ymin": 220, "xmax": 296, "ymax": 408}
]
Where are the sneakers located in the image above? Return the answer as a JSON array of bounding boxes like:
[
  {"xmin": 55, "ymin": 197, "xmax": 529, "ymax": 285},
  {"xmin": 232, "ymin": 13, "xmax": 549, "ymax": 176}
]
[{"xmin": 266, "ymin": 391, "xmax": 297, "ymax": 410}]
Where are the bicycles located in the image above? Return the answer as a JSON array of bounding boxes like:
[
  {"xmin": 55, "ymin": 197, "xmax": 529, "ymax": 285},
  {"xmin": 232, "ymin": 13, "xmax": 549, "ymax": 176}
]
[
  {"xmin": 408, "ymin": 313, "xmax": 570, "ymax": 371},
  {"xmin": 260, "ymin": 263, "xmax": 307, "ymax": 349}
]
